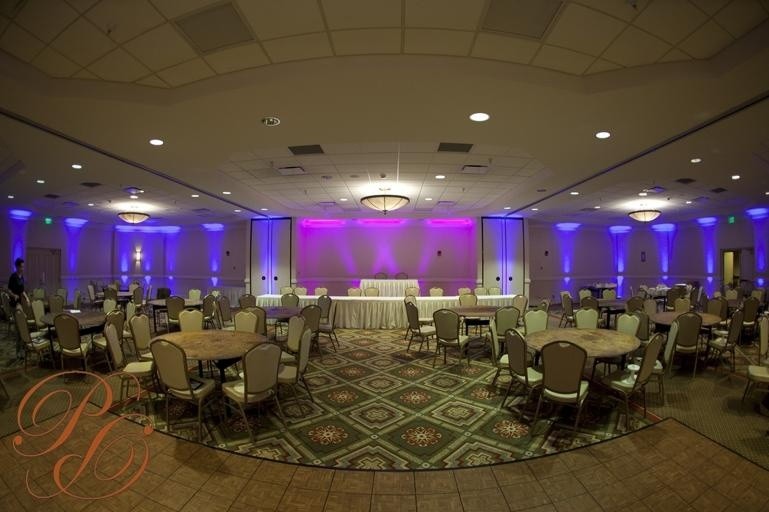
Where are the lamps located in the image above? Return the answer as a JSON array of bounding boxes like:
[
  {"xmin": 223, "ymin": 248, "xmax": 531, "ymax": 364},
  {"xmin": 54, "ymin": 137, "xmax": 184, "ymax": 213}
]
[
  {"xmin": 627, "ymin": 208, "xmax": 663, "ymax": 223},
  {"xmin": 117, "ymin": 212, "xmax": 150, "ymax": 224},
  {"xmin": 360, "ymin": 193, "xmax": 411, "ymax": 218}
]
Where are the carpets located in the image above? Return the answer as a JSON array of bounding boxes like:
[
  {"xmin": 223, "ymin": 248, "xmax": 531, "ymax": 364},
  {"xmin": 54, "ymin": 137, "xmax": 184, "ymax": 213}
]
[{"xmin": 0, "ymin": 319, "xmax": 768, "ymax": 472}]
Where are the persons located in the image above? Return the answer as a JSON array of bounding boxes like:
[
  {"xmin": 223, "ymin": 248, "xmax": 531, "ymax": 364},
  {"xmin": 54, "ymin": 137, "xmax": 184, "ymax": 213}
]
[{"xmin": 7, "ymin": 258, "xmax": 29, "ymax": 360}]
